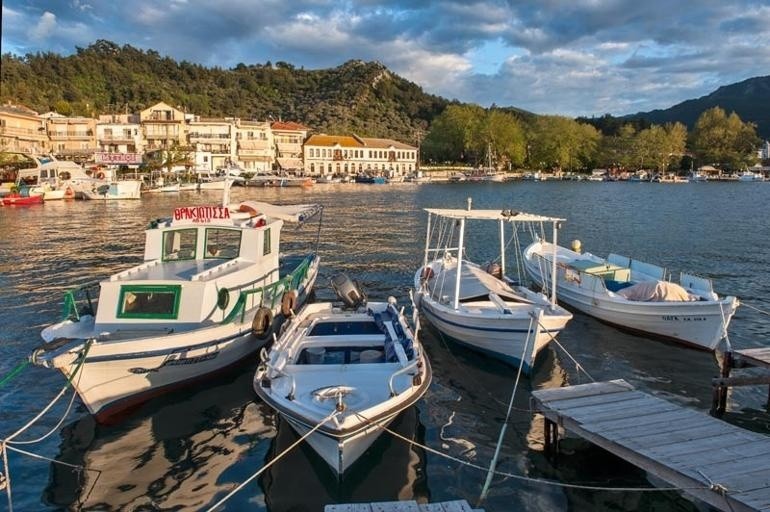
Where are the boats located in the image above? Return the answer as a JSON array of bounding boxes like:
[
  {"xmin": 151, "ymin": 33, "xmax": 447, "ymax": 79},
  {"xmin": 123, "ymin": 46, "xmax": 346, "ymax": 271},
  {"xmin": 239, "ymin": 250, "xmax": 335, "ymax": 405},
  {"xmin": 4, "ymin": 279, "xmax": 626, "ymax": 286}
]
[
  {"xmin": 0, "ymin": 180, "xmax": 74, "ymax": 204},
  {"xmin": 44, "ymin": 202, "xmax": 323, "ymax": 426},
  {"xmin": 235, "ymin": 171, "xmax": 311, "ymax": 187},
  {"xmin": 451, "ymin": 165, "xmax": 770, "ymax": 182},
  {"xmin": 413, "ymin": 204, "xmax": 574, "ymax": 375},
  {"xmin": 523, "ymin": 231, "xmax": 738, "ymax": 352},
  {"xmin": 17, "ymin": 161, "xmax": 143, "ymax": 200},
  {"xmin": 148, "ymin": 179, "xmax": 234, "ymax": 191},
  {"xmin": 253, "ymin": 272, "xmax": 434, "ymax": 479},
  {"xmin": 318, "ymin": 172, "xmax": 385, "ymax": 184}
]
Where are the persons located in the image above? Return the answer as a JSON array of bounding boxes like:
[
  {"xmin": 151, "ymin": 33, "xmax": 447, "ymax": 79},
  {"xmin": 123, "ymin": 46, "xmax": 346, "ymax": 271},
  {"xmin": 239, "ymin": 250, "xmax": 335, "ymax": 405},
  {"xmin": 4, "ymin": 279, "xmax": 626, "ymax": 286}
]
[{"xmin": 206, "ymin": 245, "xmax": 221, "ymax": 257}]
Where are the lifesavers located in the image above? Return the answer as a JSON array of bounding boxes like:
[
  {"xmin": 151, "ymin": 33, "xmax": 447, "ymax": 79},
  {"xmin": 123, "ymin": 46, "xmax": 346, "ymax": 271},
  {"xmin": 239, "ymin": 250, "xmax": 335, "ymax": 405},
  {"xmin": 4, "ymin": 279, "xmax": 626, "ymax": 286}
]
[
  {"xmin": 312, "ymin": 386, "xmax": 371, "ymax": 412},
  {"xmin": 224, "ymin": 203, "xmax": 257, "ymax": 220},
  {"xmin": 97, "ymin": 172, "xmax": 104, "ymax": 179},
  {"xmin": 420, "ymin": 267, "xmax": 434, "ymax": 285}
]
